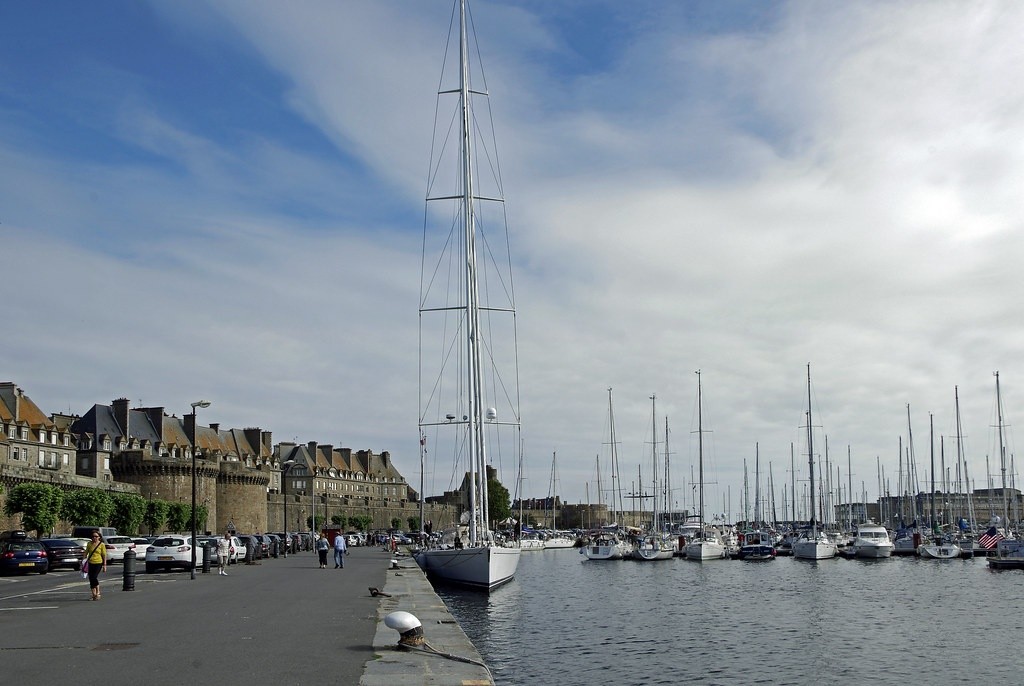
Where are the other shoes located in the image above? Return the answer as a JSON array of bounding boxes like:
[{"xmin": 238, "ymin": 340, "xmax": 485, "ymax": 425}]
[
  {"xmin": 92, "ymin": 594, "xmax": 101, "ymax": 600},
  {"xmin": 340, "ymin": 563, "xmax": 343, "ymax": 568},
  {"xmin": 335, "ymin": 565, "xmax": 339, "ymax": 569},
  {"xmin": 319, "ymin": 566, "xmax": 322, "ymax": 568},
  {"xmin": 323, "ymin": 567, "xmax": 327, "ymax": 569}
]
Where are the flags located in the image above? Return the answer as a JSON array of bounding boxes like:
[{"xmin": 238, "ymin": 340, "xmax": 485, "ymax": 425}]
[{"xmin": 978, "ymin": 526, "xmax": 1004, "ymax": 550}]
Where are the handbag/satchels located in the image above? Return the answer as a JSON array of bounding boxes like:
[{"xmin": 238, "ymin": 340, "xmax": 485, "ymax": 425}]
[
  {"xmin": 82, "ymin": 563, "xmax": 89, "ymax": 572},
  {"xmin": 344, "ymin": 550, "xmax": 350, "ymax": 556}
]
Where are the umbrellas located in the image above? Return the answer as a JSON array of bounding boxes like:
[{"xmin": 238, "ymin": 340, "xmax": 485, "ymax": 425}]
[{"xmin": 500, "ymin": 517, "xmax": 518, "ymax": 526}]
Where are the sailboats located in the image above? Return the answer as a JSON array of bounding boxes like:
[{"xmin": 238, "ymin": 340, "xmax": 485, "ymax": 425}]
[
  {"xmin": 423, "ymin": 0, "xmax": 523, "ymax": 595},
  {"xmin": 579, "ymin": 361, "xmax": 1024, "ymax": 561}
]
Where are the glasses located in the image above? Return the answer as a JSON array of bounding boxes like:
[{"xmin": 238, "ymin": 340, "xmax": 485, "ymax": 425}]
[{"xmin": 92, "ymin": 536, "xmax": 100, "ymax": 538}]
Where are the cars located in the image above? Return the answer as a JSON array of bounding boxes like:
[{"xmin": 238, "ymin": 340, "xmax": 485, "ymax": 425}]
[
  {"xmin": 196, "ymin": 531, "xmax": 320, "ymax": 564},
  {"xmin": 145, "ymin": 534, "xmax": 203, "ymax": 574},
  {"xmin": 0, "ymin": 526, "xmax": 157, "ymax": 574},
  {"xmin": 342, "ymin": 530, "xmax": 443, "ymax": 547}
]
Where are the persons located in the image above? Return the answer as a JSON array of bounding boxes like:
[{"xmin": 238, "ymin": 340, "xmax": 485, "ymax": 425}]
[
  {"xmin": 367, "ymin": 532, "xmax": 382, "ymax": 547},
  {"xmin": 215, "ymin": 532, "xmax": 231, "ymax": 576},
  {"xmin": 316, "ymin": 533, "xmax": 330, "ymax": 569},
  {"xmin": 82, "ymin": 532, "xmax": 107, "ymax": 601},
  {"xmin": 334, "ymin": 532, "xmax": 350, "ymax": 569}
]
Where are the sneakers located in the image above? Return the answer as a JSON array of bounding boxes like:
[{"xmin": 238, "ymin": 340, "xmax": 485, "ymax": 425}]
[
  {"xmin": 218, "ymin": 571, "xmax": 222, "ymax": 574},
  {"xmin": 222, "ymin": 571, "xmax": 228, "ymax": 576}
]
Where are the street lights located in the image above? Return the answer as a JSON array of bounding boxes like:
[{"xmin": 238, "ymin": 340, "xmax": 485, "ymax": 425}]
[
  {"xmin": 190, "ymin": 400, "xmax": 211, "ymax": 580},
  {"xmin": 283, "ymin": 460, "xmax": 295, "ymax": 558}
]
